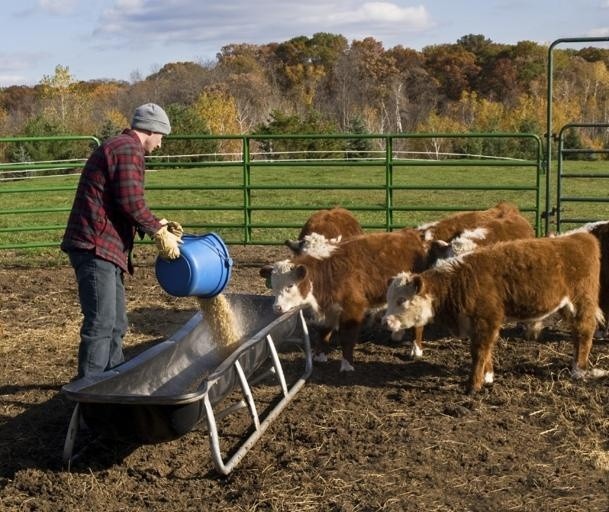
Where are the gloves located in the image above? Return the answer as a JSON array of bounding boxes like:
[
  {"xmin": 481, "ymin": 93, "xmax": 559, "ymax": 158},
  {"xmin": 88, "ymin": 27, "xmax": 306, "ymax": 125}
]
[
  {"xmin": 158, "ymin": 219, "xmax": 184, "ymax": 238},
  {"xmin": 150, "ymin": 224, "xmax": 185, "ymax": 260}
]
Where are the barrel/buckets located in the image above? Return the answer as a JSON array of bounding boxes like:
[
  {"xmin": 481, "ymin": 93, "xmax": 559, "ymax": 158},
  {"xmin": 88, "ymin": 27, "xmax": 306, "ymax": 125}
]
[{"xmin": 154, "ymin": 231, "xmax": 233, "ymax": 299}]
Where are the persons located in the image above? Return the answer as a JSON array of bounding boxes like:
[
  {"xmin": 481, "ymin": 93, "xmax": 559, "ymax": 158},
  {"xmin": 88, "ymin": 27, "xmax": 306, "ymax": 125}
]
[{"xmin": 60, "ymin": 102, "xmax": 184, "ymax": 441}]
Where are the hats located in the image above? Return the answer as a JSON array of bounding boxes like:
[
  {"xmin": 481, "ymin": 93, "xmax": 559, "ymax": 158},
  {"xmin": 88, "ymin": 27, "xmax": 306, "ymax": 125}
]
[{"xmin": 131, "ymin": 103, "xmax": 172, "ymax": 135}]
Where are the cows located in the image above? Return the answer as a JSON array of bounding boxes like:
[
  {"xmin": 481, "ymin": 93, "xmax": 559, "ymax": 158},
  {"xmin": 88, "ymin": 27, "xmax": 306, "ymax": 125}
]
[
  {"xmin": 420, "ymin": 202, "xmax": 542, "ymax": 262},
  {"xmin": 284, "ymin": 205, "xmax": 363, "ymax": 256},
  {"xmin": 381, "ymin": 230, "xmax": 602, "ymax": 396},
  {"xmin": 262, "ymin": 228, "xmax": 423, "ymax": 371},
  {"xmin": 549, "ymin": 220, "xmax": 609, "ymax": 339}
]
